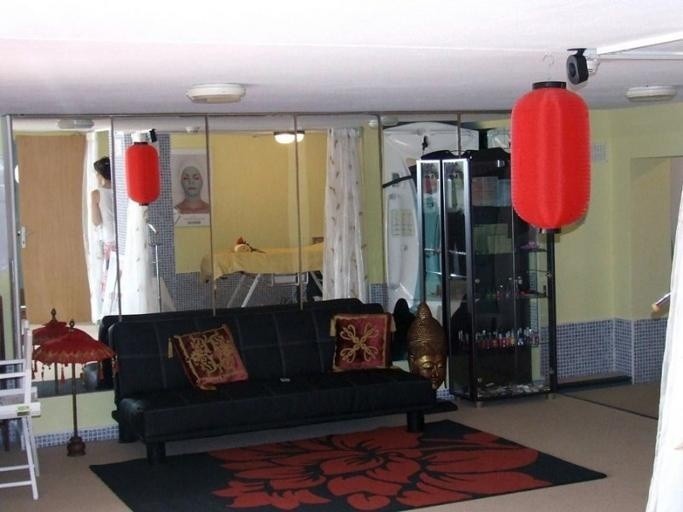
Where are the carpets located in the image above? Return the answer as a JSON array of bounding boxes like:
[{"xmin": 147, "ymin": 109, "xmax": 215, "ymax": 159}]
[{"xmin": 87, "ymin": 418, "xmax": 608, "ymax": 512}]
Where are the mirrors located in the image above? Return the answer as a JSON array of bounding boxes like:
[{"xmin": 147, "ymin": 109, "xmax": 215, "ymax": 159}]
[
  {"xmin": 459, "ymin": 109, "xmax": 511, "ymax": 157},
  {"xmin": 207, "ymin": 112, "xmax": 301, "ymax": 310},
  {"xmin": 294, "ymin": 112, "xmax": 385, "ymax": 310},
  {"xmin": 378, "ymin": 107, "xmax": 459, "ymax": 309},
  {"xmin": 112, "ymin": 114, "xmax": 215, "ymax": 320},
  {"xmin": 4, "ymin": 112, "xmax": 121, "ymax": 399}
]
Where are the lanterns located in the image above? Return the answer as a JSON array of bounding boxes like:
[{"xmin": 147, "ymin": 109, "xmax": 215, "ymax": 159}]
[
  {"xmin": 124, "ymin": 134, "xmax": 161, "ymax": 208},
  {"xmin": 510, "ymin": 81, "xmax": 591, "ymax": 234}
]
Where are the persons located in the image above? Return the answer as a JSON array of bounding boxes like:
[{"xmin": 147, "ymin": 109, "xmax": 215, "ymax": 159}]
[
  {"xmin": 90, "ymin": 157, "xmax": 116, "ymax": 261},
  {"xmin": 406, "ymin": 302, "xmax": 446, "ymax": 390},
  {"xmin": 173, "ymin": 160, "xmax": 210, "ymax": 224}
]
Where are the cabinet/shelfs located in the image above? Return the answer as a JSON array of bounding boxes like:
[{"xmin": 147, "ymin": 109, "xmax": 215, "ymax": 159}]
[
  {"xmin": 442, "ymin": 153, "xmax": 559, "ymax": 409},
  {"xmin": 414, "ymin": 157, "xmax": 443, "ymax": 332}
]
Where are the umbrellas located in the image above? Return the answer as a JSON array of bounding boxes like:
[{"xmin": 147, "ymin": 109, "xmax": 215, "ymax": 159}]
[
  {"xmin": 31, "ymin": 318, "xmax": 114, "ymax": 457},
  {"xmin": 32, "ymin": 307, "xmax": 68, "ymax": 396}
]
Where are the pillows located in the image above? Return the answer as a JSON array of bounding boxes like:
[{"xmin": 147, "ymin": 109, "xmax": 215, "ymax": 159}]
[
  {"xmin": 167, "ymin": 324, "xmax": 249, "ymax": 391},
  {"xmin": 328, "ymin": 312, "xmax": 391, "ymax": 372}
]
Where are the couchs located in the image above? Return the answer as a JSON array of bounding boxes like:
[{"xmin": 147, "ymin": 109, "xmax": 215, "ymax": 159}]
[
  {"xmin": 78, "ymin": 296, "xmax": 365, "ymax": 394},
  {"xmin": 108, "ymin": 302, "xmax": 434, "ymax": 458}
]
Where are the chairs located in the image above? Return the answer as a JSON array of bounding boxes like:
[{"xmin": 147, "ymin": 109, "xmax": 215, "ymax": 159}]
[{"xmin": 0, "ymin": 328, "xmax": 42, "ymax": 500}]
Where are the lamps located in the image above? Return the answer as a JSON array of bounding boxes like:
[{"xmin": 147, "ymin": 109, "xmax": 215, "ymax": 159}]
[
  {"xmin": 296, "ymin": 130, "xmax": 305, "ymax": 143},
  {"xmin": 273, "ymin": 132, "xmax": 295, "ymax": 145}
]
